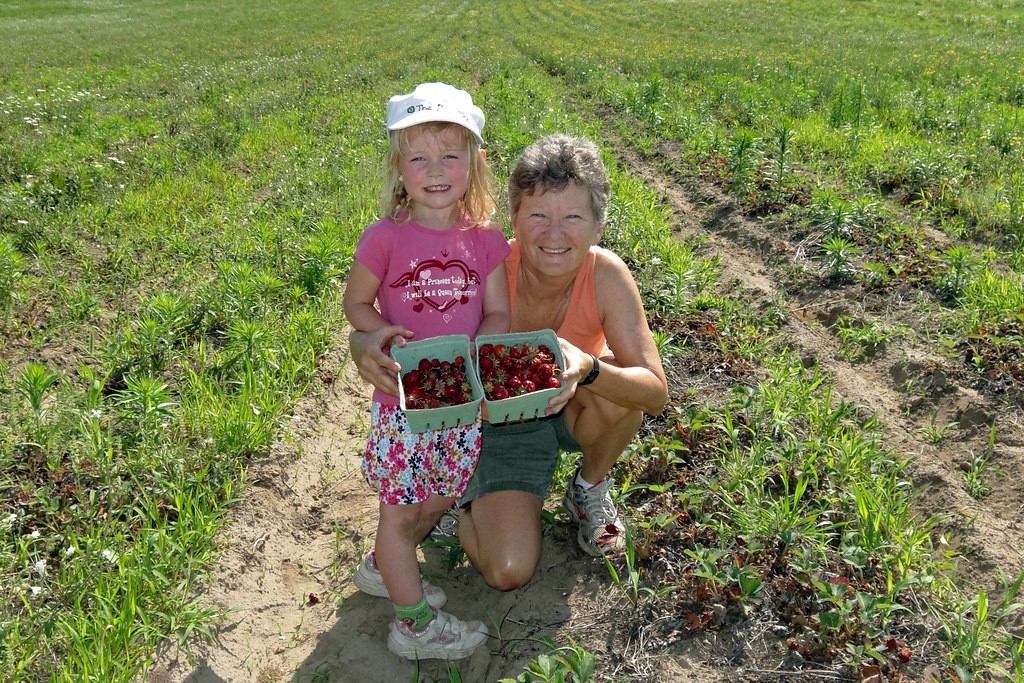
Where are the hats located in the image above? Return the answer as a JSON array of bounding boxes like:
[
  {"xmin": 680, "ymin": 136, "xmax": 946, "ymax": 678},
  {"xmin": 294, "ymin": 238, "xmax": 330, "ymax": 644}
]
[{"xmin": 387, "ymin": 82, "xmax": 485, "ymax": 146}]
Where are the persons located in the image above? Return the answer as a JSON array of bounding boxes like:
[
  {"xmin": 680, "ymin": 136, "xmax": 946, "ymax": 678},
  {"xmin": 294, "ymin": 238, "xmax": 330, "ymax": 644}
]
[
  {"xmin": 344, "ymin": 81, "xmax": 511, "ymax": 659},
  {"xmin": 348, "ymin": 134, "xmax": 669, "ymax": 593}
]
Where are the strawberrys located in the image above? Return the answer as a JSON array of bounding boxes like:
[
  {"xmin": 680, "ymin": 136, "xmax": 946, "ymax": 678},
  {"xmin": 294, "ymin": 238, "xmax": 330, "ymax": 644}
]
[
  {"xmin": 402, "ymin": 355, "xmax": 475, "ymax": 410},
  {"xmin": 478, "ymin": 344, "xmax": 561, "ymax": 401}
]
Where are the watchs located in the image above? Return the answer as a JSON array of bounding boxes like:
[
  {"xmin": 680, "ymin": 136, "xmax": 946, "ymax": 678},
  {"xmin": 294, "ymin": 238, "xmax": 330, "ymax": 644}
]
[{"xmin": 578, "ymin": 354, "xmax": 600, "ymax": 387}]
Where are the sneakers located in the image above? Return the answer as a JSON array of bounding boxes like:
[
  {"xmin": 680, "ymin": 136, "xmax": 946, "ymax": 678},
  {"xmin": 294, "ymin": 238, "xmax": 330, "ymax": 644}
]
[
  {"xmin": 387, "ymin": 608, "xmax": 489, "ymax": 660},
  {"xmin": 354, "ymin": 548, "xmax": 448, "ymax": 609},
  {"xmin": 563, "ymin": 467, "xmax": 627, "ymax": 557},
  {"xmin": 431, "ymin": 497, "xmax": 463, "ymax": 550}
]
